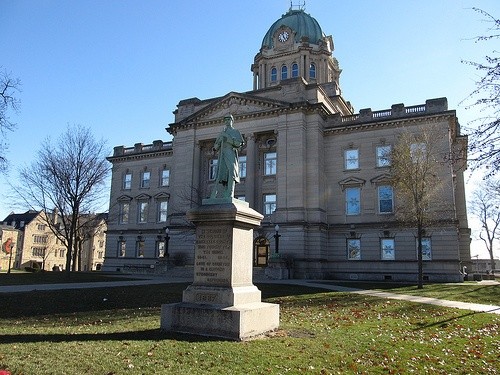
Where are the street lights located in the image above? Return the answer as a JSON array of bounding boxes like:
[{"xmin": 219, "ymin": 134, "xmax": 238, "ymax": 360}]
[
  {"xmin": 271, "ymin": 224, "xmax": 282, "ymax": 257},
  {"xmin": 8, "ymin": 243, "xmax": 15, "ymax": 273},
  {"xmin": 162, "ymin": 228, "xmax": 171, "ymax": 258}
]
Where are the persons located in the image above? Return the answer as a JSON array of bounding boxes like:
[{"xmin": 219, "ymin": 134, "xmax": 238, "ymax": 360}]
[
  {"xmin": 96, "ymin": 262, "xmax": 102, "ymax": 271},
  {"xmin": 208, "ymin": 112, "xmax": 246, "ymax": 198},
  {"xmin": 52, "ymin": 264, "xmax": 59, "ymax": 272},
  {"xmin": 58, "ymin": 264, "xmax": 63, "ymax": 272}
]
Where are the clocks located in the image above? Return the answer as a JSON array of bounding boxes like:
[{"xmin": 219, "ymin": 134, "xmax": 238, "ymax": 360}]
[{"xmin": 278, "ymin": 31, "xmax": 289, "ymax": 43}]
